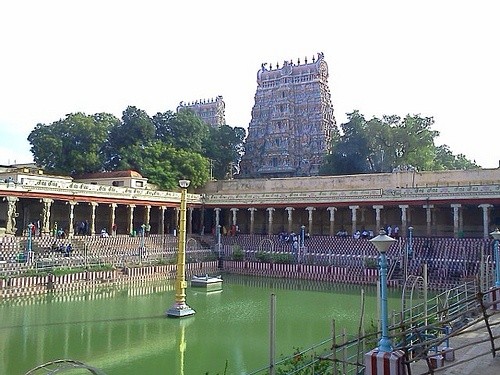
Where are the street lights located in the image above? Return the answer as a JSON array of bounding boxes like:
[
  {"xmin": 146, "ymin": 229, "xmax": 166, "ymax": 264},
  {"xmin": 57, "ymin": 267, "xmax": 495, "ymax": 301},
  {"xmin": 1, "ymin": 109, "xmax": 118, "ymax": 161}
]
[
  {"xmin": 490, "ymin": 227, "xmax": 500, "ymax": 287},
  {"xmin": 300, "ymin": 225, "xmax": 306, "ymax": 247},
  {"xmin": 217, "ymin": 225, "xmax": 221, "ymax": 259},
  {"xmin": 140, "ymin": 223, "xmax": 147, "ymax": 247},
  {"xmin": 28, "ymin": 223, "xmax": 34, "ymax": 251},
  {"xmin": 367, "ymin": 226, "xmax": 398, "ymax": 353},
  {"xmin": 407, "ymin": 226, "xmax": 414, "ymax": 249}
]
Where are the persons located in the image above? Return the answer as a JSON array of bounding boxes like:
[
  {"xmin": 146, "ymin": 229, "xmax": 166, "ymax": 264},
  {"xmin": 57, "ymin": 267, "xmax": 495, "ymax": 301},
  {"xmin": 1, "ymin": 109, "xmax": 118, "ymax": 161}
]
[{"xmin": 27, "ymin": 217, "xmax": 400, "ymax": 255}]
